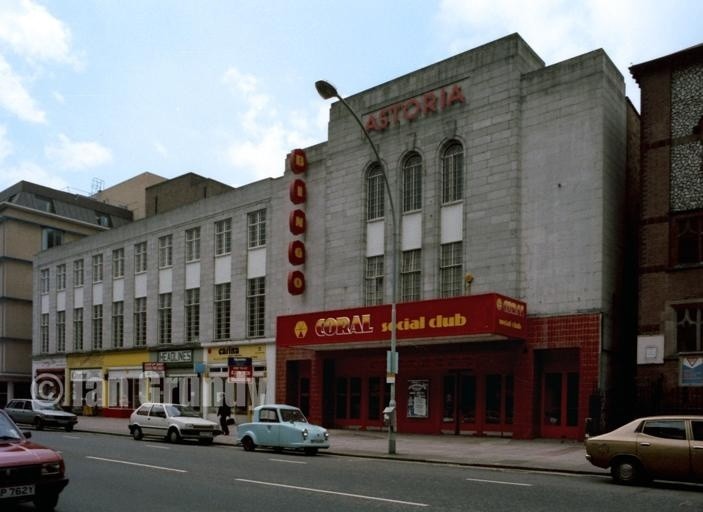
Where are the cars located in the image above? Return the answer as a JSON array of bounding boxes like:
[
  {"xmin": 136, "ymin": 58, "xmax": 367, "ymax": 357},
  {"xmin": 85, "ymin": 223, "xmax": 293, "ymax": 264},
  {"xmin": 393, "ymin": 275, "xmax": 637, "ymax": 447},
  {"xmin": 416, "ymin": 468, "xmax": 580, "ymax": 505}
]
[
  {"xmin": 583, "ymin": 415, "xmax": 702, "ymax": 485},
  {"xmin": 3, "ymin": 399, "xmax": 78, "ymax": 431},
  {"xmin": 234, "ymin": 403, "xmax": 329, "ymax": 455},
  {"xmin": 0, "ymin": 408, "xmax": 68, "ymax": 508},
  {"xmin": 127, "ymin": 402, "xmax": 219, "ymax": 445}
]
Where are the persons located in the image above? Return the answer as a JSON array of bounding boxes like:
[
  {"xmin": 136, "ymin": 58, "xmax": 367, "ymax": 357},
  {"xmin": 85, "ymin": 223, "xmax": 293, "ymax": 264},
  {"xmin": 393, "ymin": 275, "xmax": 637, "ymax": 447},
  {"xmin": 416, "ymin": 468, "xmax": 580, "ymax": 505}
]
[{"xmin": 217, "ymin": 396, "xmax": 232, "ymax": 435}]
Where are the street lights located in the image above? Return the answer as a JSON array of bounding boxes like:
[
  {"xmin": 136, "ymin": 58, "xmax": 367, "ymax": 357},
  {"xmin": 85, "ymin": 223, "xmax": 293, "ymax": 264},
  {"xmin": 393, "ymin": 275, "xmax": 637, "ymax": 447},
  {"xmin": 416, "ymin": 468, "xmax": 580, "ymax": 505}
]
[{"xmin": 315, "ymin": 81, "xmax": 396, "ymax": 454}]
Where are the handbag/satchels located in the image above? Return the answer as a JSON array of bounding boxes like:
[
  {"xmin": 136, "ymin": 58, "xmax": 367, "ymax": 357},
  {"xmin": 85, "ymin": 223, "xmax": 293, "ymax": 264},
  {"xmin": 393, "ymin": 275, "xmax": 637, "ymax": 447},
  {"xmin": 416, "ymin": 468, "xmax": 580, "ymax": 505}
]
[{"xmin": 226, "ymin": 418, "xmax": 235, "ymax": 426}]
